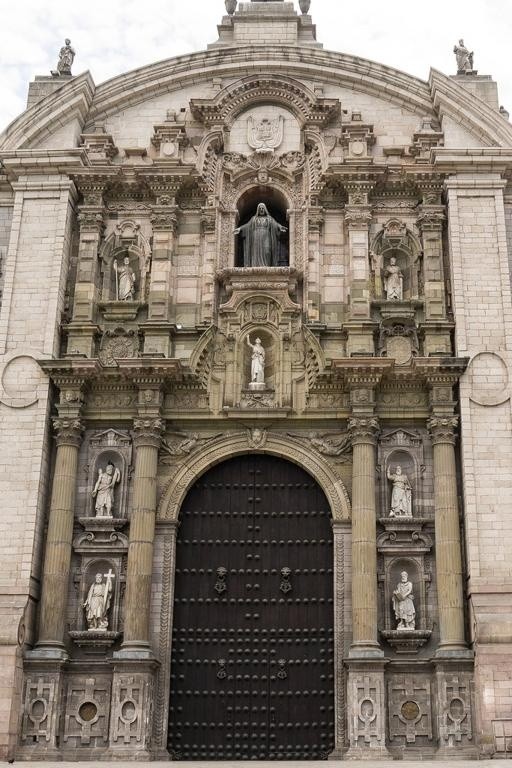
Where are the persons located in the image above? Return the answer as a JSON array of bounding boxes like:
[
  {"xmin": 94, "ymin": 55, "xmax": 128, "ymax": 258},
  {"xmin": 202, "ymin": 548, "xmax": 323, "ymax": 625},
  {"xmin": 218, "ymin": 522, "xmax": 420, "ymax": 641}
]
[
  {"xmin": 385, "ymin": 461, "xmax": 414, "ymax": 519},
  {"xmin": 231, "ymin": 201, "xmax": 289, "ymax": 268},
  {"xmin": 88, "ymin": 464, "xmax": 121, "ymax": 519},
  {"xmin": 383, "ymin": 256, "xmax": 406, "ymax": 301},
  {"xmin": 80, "ymin": 572, "xmax": 114, "ymax": 633},
  {"xmin": 390, "ymin": 570, "xmax": 417, "ymax": 631},
  {"xmin": 245, "ymin": 331, "xmax": 266, "ymax": 384},
  {"xmin": 112, "ymin": 255, "xmax": 137, "ymax": 303},
  {"xmin": 452, "ymin": 37, "xmax": 478, "ymax": 77},
  {"xmin": 56, "ymin": 38, "xmax": 76, "ymax": 77}
]
